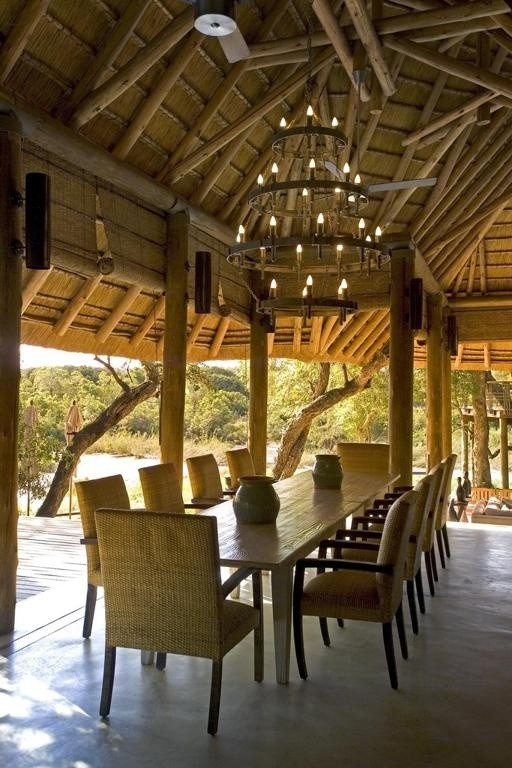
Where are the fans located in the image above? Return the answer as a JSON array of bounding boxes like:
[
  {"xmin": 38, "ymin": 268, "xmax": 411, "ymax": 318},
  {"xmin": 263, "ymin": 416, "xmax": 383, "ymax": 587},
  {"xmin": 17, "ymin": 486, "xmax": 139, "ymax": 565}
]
[
  {"xmin": 318, "ymin": 69, "xmax": 437, "ymax": 193},
  {"xmin": 182, "ymin": 0, "xmax": 250, "ymax": 64}
]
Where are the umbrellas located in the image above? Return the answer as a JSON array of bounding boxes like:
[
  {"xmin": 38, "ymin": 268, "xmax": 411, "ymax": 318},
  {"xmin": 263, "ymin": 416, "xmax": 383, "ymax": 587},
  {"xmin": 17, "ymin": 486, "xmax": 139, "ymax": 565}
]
[
  {"xmin": 65, "ymin": 400, "xmax": 82, "ymax": 448},
  {"xmin": 23, "ymin": 400, "xmax": 38, "ymax": 476}
]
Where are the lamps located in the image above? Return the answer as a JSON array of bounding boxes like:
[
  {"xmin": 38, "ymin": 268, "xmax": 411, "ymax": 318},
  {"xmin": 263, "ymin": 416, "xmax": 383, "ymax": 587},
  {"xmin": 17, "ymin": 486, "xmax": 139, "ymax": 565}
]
[
  {"xmin": 184, "ymin": 251, "xmax": 211, "ymax": 314},
  {"xmin": 448, "ymin": 316, "xmax": 456, "ymax": 354},
  {"xmin": 9, "ymin": 172, "xmax": 51, "ymax": 270},
  {"xmin": 225, "ymin": 11, "xmax": 393, "ymax": 325},
  {"xmin": 410, "ymin": 278, "xmax": 422, "ymax": 330}
]
